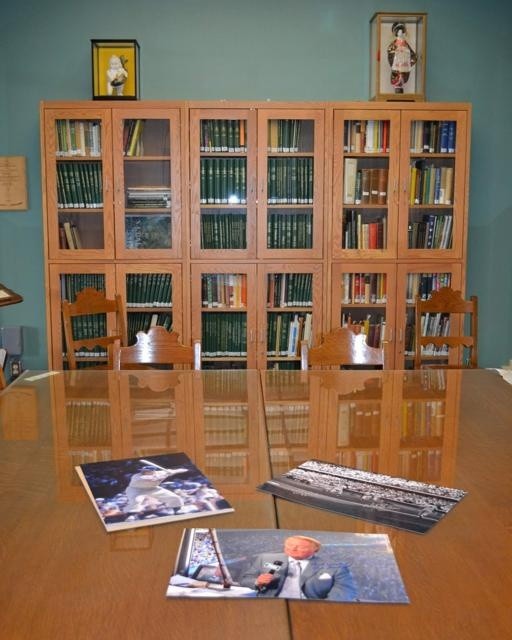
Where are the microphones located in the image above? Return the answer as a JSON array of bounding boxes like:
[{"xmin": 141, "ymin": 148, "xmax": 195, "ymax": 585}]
[{"xmin": 259, "ymin": 558, "xmax": 283, "ymax": 592}]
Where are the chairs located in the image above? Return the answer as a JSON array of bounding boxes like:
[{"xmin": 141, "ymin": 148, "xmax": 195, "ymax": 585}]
[
  {"xmin": 61, "ymin": 286, "xmax": 128, "ymax": 369},
  {"xmin": 109, "ymin": 325, "xmax": 202, "ymax": 370},
  {"xmin": 414, "ymin": 283, "xmax": 479, "ymax": 369},
  {"xmin": 300, "ymin": 324, "xmax": 391, "ymax": 369}
]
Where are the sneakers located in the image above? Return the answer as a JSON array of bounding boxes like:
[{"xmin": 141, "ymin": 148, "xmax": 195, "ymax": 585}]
[
  {"xmin": 124, "ymin": 514, "xmax": 139, "ymax": 522},
  {"xmin": 173, "ymin": 508, "xmax": 188, "ymax": 516}
]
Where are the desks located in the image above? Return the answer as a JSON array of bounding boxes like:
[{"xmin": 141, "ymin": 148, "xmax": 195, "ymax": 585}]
[
  {"xmin": 260, "ymin": 368, "xmax": 512, "ymax": 640},
  {"xmin": 0, "ymin": 368, "xmax": 292, "ymax": 640}
]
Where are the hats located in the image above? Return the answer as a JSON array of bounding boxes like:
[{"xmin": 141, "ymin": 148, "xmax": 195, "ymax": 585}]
[{"xmin": 137, "ymin": 466, "xmax": 158, "ymax": 472}]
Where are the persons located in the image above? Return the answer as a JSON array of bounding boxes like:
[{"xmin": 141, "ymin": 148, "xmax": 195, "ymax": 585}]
[
  {"xmin": 239, "ymin": 534, "xmax": 358, "ymax": 602},
  {"xmin": 106, "ymin": 54, "xmax": 128, "ymax": 96},
  {"xmin": 94, "ymin": 462, "xmax": 224, "ymax": 523},
  {"xmin": 387, "ymin": 22, "xmax": 418, "ymax": 94}
]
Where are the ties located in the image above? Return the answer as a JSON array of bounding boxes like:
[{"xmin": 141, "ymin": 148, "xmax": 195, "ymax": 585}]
[{"xmin": 291, "ymin": 561, "xmax": 302, "ymax": 597}]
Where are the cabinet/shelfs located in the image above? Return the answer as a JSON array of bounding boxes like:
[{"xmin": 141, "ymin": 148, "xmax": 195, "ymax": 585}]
[
  {"xmin": 42, "ymin": 262, "xmax": 186, "ymax": 369},
  {"xmin": 44, "ymin": 369, "xmax": 463, "ymax": 505},
  {"xmin": 186, "ymin": 100, "xmax": 328, "ymax": 262},
  {"xmin": 329, "ymin": 101, "xmax": 473, "ymax": 260},
  {"xmin": 185, "ymin": 262, "xmax": 327, "ymax": 371},
  {"xmin": 39, "ymin": 101, "xmax": 186, "ymax": 263},
  {"xmin": 326, "ymin": 261, "xmax": 467, "ymax": 369}
]
